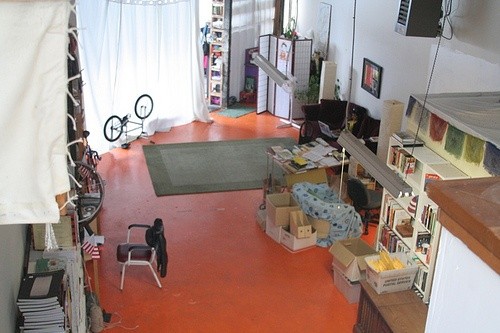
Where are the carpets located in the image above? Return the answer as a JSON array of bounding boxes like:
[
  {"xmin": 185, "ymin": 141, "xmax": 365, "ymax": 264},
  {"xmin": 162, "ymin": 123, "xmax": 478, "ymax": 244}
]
[
  {"xmin": 218, "ymin": 103, "xmax": 257, "ymax": 118},
  {"xmin": 143, "ymin": 136, "xmax": 298, "ymax": 196}
]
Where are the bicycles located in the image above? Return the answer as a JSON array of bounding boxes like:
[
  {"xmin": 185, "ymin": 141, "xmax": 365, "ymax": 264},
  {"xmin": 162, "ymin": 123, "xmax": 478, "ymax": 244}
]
[{"xmin": 103, "ymin": 94, "xmax": 156, "ymax": 150}]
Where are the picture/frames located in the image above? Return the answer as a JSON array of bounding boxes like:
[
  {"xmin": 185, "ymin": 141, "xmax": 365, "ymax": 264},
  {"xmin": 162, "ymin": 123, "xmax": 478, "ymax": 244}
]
[{"xmin": 361, "ymin": 57, "xmax": 383, "ymax": 99}]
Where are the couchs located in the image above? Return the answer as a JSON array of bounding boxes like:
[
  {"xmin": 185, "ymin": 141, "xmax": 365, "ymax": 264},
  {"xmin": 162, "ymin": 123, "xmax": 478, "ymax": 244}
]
[{"xmin": 301, "ymin": 99, "xmax": 380, "ymax": 172}]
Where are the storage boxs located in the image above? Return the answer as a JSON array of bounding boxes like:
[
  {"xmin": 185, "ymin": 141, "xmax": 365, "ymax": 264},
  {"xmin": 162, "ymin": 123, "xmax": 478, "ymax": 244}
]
[
  {"xmin": 256, "ymin": 167, "xmax": 330, "ymax": 253},
  {"xmin": 331, "ymin": 262, "xmax": 362, "ymax": 305},
  {"xmin": 240, "ymin": 91, "xmax": 257, "ymax": 108},
  {"xmin": 363, "ymin": 251, "xmax": 418, "ymax": 295},
  {"xmin": 326, "ymin": 237, "xmax": 379, "ymax": 283}
]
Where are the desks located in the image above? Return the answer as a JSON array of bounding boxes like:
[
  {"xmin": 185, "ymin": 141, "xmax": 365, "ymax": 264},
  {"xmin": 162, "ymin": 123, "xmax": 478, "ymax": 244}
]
[
  {"xmin": 83, "ymin": 209, "xmax": 98, "ymax": 296},
  {"xmin": 265, "ymin": 139, "xmax": 352, "ymax": 203},
  {"xmin": 352, "ymin": 277, "xmax": 427, "ymax": 333}
]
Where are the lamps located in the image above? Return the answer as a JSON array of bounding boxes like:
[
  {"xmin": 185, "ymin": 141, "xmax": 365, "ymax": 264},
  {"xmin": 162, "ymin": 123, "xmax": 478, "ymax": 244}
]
[
  {"xmin": 337, "ymin": 0, "xmax": 453, "ymax": 197},
  {"xmin": 253, "ymin": 0, "xmax": 300, "ymax": 91}
]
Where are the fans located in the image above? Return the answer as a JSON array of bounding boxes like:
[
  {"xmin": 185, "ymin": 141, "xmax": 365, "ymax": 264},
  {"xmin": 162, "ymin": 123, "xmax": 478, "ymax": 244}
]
[{"xmin": 277, "ymin": 75, "xmax": 303, "ymax": 131}]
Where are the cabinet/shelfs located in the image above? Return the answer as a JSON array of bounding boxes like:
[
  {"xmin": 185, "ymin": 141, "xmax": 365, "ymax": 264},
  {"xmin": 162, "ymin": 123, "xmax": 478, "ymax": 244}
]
[
  {"xmin": 208, "ymin": 0, "xmax": 226, "ymax": 108},
  {"xmin": 377, "ymin": 136, "xmax": 469, "ymax": 305}
]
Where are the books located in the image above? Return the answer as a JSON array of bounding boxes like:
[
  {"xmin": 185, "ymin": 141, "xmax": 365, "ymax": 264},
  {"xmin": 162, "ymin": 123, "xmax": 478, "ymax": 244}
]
[
  {"xmin": 391, "ymin": 132, "xmax": 424, "ymax": 146},
  {"xmin": 16, "ymin": 269, "xmax": 70, "ymax": 333},
  {"xmin": 424, "ymin": 174, "xmax": 439, "ymax": 192},
  {"xmin": 268, "ymin": 139, "xmax": 344, "ymax": 171},
  {"xmin": 378, "ymin": 194, "xmax": 437, "ymax": 299},
  {"xmin": 390, "ymin": 146, "xmax": 415, "ymax": 173}
]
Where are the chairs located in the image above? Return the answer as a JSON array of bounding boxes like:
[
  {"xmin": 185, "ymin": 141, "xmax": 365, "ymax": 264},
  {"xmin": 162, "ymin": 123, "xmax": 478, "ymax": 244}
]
[
  {"xmin": 349, "ymin": 178, "xmax": 382, "ymax": 234},
  {"xmin": 117, "ymin": 218, "xmax": 163, "ymax": 289}
]
